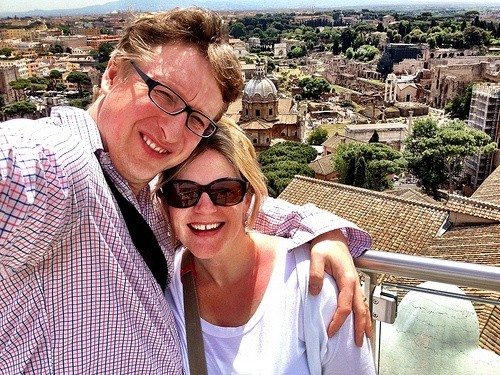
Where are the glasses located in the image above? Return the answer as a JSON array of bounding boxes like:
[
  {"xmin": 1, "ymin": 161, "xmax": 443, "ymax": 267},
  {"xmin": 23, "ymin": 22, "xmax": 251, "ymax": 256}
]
[
  {"xmin": 156, "ymin": 177, "xmax": 251, "ymax": 208},
  {"xmin": 130, "ymin": 60, "xmax": 220, "ymax": 138}
]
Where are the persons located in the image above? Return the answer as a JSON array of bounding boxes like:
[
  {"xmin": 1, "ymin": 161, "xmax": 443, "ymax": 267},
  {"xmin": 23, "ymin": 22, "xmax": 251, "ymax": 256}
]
[
  {"xmin": 157, "ymin": 114, "xmax": 373, "ymax": 375},
  {"xmin": 0, "ymin": 7, "xmax": 375, "ymax": 375}
]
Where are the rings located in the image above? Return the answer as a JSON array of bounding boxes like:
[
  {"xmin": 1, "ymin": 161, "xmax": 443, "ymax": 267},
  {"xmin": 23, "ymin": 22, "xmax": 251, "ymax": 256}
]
[{"xmin": 363, "ymin": 298, "xmax": 366, "ymax": 302}]
